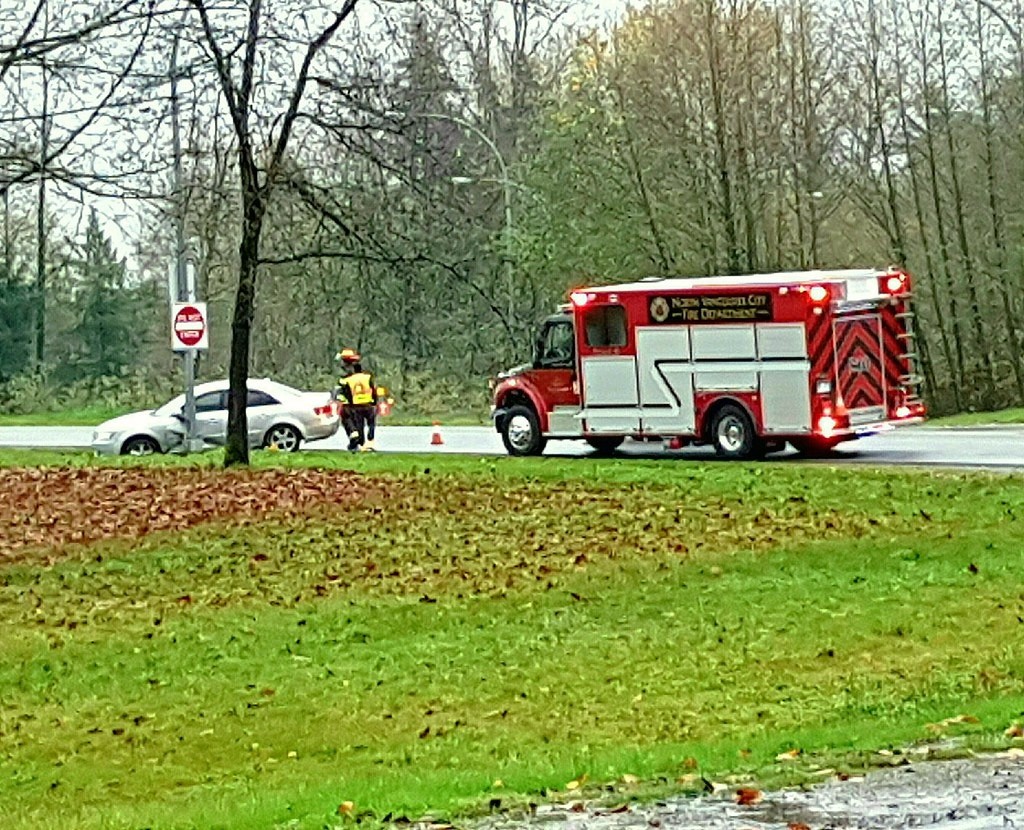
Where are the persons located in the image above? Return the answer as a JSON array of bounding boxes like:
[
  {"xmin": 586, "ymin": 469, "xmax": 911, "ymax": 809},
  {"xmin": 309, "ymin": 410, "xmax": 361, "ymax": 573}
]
[{"xmin": 334, "ymin": 349, "xmax": 379, "ymax": 452}]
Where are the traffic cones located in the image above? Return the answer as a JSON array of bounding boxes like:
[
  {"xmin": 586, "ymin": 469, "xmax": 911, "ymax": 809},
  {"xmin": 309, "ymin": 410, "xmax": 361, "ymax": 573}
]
[{"xmin": 430, "ymin": 417, "xmax": 444, "ymax": 444}]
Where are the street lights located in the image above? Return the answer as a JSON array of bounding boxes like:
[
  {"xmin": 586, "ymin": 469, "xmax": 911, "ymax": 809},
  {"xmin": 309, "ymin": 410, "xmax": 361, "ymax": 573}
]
[{"xmin": 417, "ymin": 110, "xmax": 514, "ymax": 371}]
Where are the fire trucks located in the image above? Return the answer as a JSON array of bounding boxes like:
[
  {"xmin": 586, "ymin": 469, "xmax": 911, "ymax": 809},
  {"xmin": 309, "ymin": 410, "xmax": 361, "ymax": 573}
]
[{"xmin": 490, "ymin": 267, "xmax": 931, "ymax": 459}]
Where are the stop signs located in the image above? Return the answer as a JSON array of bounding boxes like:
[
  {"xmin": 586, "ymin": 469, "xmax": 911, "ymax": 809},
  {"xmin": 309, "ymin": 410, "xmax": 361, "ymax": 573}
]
[{"xmin": 170, "ymin": 300, "xmax": 208, "ymax": 351}]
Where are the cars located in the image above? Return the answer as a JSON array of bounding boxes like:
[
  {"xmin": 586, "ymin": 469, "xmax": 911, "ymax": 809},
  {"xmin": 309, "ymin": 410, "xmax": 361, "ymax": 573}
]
[{"xmin": 88, "ymin": 373, "xmax": 338, "ymax": 460}]
